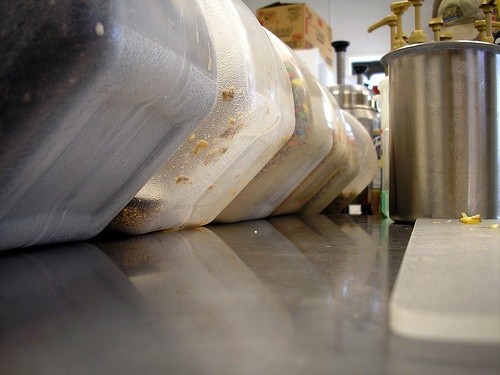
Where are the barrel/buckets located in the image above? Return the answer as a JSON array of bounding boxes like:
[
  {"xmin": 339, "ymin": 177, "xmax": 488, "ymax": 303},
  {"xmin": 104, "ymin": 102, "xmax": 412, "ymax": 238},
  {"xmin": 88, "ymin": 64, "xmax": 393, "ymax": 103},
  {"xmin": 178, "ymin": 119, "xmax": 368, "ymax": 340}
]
[{"xmin": 379, "ymin": 39, "xmax": 500, "ymax": 223}]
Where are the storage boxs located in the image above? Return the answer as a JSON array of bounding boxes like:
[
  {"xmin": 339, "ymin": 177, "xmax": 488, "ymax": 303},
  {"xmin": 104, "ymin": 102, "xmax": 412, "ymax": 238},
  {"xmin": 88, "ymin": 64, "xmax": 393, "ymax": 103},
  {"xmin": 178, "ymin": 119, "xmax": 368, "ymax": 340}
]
[
  {"xmin": 295, "ymin": 46, "xmax": 339, "ymax": 87},
  {"xmin": 256, "ymin": 2, "xmax": 333, "ymax": 50}
]
